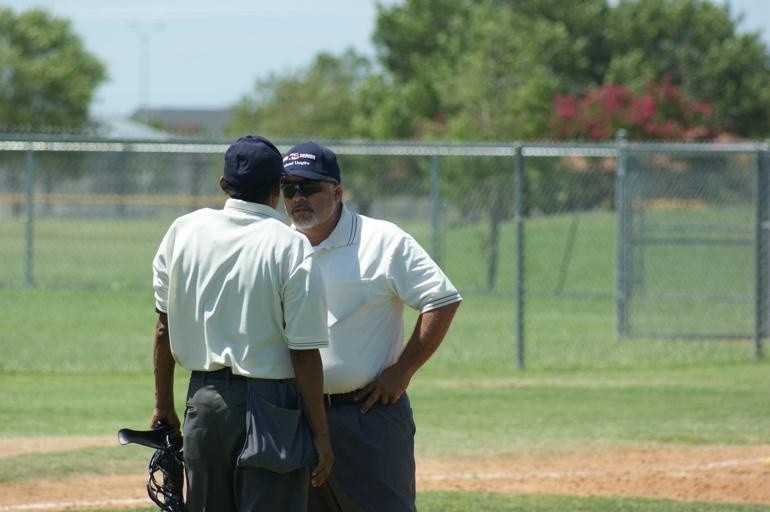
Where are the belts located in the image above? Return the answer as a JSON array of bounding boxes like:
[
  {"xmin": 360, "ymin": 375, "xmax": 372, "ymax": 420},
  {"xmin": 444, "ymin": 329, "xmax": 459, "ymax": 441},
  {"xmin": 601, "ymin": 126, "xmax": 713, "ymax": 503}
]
[
  {"xmin": 192, "ymin": 369, "xmax": 245, "ymax": 379},
  {"xmin": 324, "ymin": 389, "xmax": 363, "ymax": 405}
]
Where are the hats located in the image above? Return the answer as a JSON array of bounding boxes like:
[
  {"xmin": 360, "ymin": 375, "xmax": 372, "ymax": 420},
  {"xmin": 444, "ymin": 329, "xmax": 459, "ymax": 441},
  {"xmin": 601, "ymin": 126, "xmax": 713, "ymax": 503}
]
[
  {"xmin": 283, "ymin": 142, "xmax": 340, "ymax": 182},
  {"xmin": 224, "ymin": 136, "xmax": 289, "ymax": 185}
]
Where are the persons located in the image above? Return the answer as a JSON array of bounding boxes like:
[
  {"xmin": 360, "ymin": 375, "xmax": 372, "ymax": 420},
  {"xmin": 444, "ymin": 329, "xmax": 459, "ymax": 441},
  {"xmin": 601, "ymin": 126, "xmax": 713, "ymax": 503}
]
[
  {"xmin": 280, "ymin": 141, "xmax": 463, "ymax": 512},
  {"xmin": 150, "ymin": 135, "xmax": 337, "ymax": 512}
]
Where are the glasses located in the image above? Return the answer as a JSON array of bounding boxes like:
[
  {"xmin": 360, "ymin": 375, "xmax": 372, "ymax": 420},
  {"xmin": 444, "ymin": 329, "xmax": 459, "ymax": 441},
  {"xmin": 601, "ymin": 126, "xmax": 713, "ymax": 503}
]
[{"xmin": 284, "ymin": 180, "xmax": 322, "ymax": 198}]
